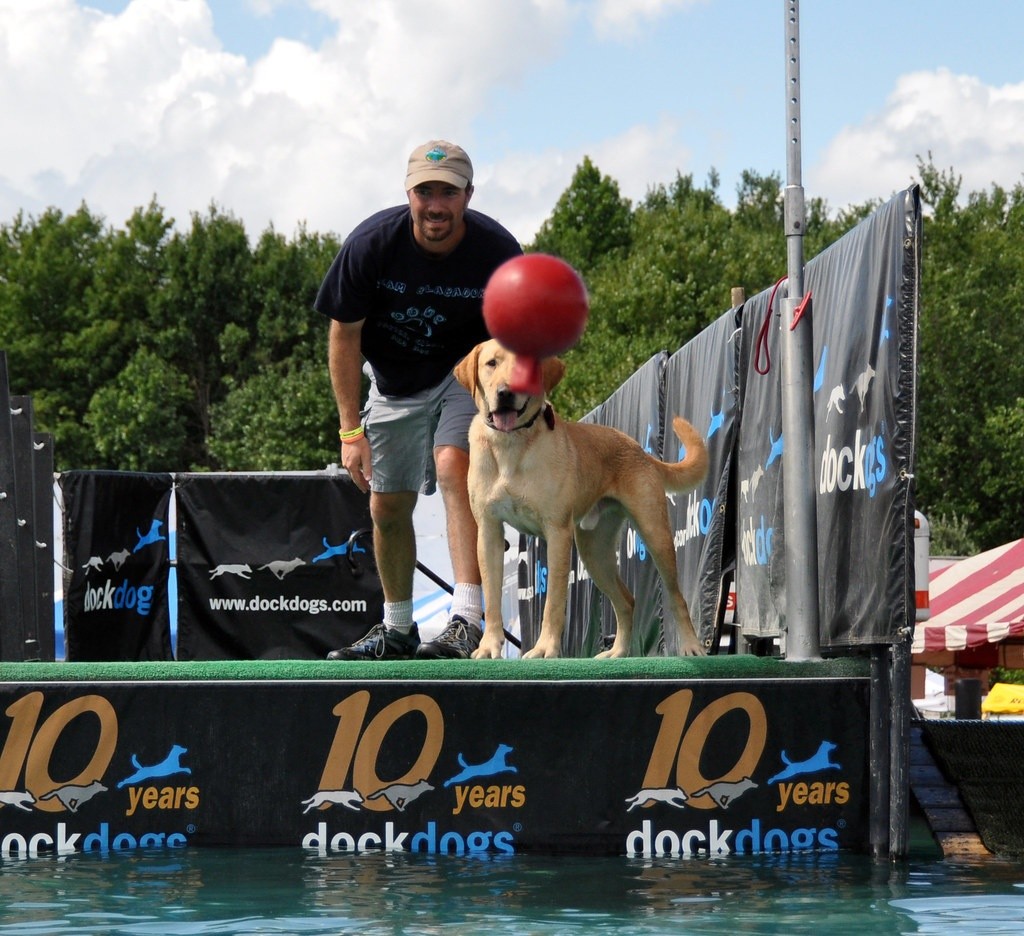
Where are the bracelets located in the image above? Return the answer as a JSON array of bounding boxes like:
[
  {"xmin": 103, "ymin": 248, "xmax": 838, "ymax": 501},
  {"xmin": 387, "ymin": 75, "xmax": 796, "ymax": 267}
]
[{"xmin": 338, "ymin": 425, "xmax": 368, "ymax": 442}]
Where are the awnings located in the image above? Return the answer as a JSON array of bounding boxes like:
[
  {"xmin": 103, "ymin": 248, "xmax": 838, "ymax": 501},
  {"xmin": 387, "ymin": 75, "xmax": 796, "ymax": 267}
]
[{"xmin": 910, "ymin": 537, "xmax": 1023, "ymax": 654}]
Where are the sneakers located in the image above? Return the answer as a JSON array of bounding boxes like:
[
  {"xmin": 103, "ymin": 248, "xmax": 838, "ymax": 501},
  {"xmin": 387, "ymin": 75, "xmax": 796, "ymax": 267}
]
[
  {"xmin": 415, "ymin": 613, "xmax": 483, "ymax": 660},
  {"xmin": 327, "ymin": 621, "xmax": 421, "ymax": 660}
]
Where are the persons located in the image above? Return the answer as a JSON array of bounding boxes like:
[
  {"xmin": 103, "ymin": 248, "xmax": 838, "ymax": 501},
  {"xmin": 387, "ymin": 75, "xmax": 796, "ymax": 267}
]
[{"xmin": 315, "ymin": 140, "xmax": 526, "ymax": 660}]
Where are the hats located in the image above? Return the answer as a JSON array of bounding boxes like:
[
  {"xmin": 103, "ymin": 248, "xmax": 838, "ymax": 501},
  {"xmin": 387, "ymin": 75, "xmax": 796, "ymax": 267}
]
[{"xmin": 405, "ymin": 140, "xmax": 473, "ymax": 192}]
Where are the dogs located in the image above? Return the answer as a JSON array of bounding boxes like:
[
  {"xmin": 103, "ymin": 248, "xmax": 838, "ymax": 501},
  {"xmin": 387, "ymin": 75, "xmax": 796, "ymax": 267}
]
[{"xmin": 454, "ymin": 338, "xmax": 710, "ymax": 658}]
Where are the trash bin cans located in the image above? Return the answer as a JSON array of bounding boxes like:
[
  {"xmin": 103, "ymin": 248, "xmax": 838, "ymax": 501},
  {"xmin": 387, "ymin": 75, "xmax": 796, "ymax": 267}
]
[{"xmin": 955, "ymin": 678, "xmax": 982, "ymax": 720}]
